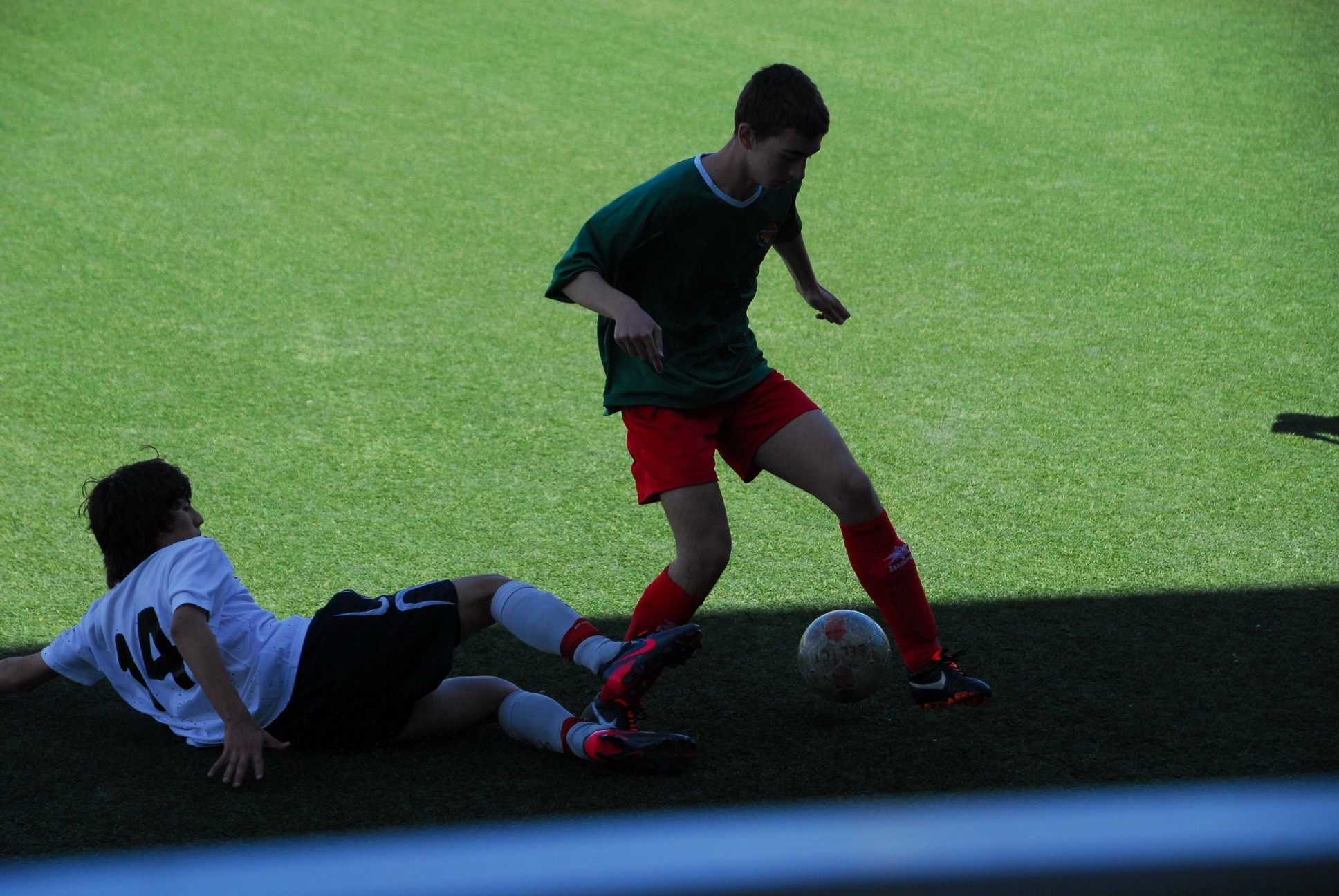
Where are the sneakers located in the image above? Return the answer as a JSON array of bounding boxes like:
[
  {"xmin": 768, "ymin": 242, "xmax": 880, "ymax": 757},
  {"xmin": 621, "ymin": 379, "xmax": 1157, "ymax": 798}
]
[
  {"xmin": 600, "ymin": 623, "xmax": 704, "ymax": 706},
  {"xmin": 580, "ymin": 695, "xmax": 640, "ymax": 733},
  {"xmin": 583, "ymin": 725, "xmax": 697, "ymax": 774},
  {"xmin": 906, "ymin": 656, "xmax": 991, "ymax": 710}
]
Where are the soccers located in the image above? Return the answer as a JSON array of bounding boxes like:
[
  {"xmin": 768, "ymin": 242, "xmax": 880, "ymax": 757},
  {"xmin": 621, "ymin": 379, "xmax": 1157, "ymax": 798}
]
[{"xmin": 797, "ymin": 610, "xmax": 892, "ymax": 705}]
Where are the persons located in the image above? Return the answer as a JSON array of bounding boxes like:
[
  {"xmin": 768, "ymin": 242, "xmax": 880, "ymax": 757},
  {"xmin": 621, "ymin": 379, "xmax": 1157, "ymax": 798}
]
[
  {"xmin": 546, "ymin": 61, "xmax": 995, "ymax": 732},
  {"xmin": 0, "ymin": 448, "xmax": 703, "ymax": 787}
]
